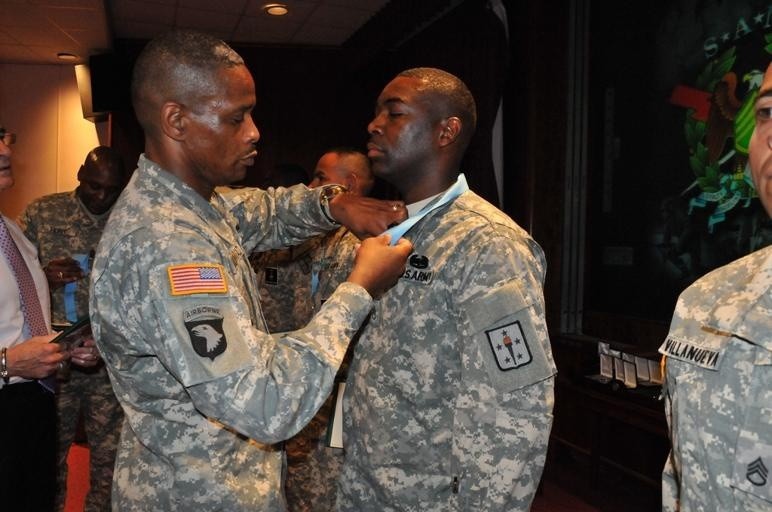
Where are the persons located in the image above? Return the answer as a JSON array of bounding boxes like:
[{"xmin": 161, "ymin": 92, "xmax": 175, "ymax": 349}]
[
  {"xmin": 661, "ymin": 59, "xmax": 772, "ymax": 512},
  {"xmin": 89, "ymin": 25, "xmax": 413, "ymax": 512},
  {"xmin": 16, "ymin": 145, "xmax": 125, "ymax": 511},
  {"xmin": 247, "ymin": 149, "xmax": 375, "ymax": 512},
  {"xmin": 332, "ymin": 68, "xmax": 557, "ymax": 512},
  {"xmin": 0, "ymin": 127, "xmax": 101, "ymax": 512}
]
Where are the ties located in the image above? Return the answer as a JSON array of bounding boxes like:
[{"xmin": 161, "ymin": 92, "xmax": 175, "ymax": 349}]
[
  {"xmin": 376, "ymin": 171, "xmax": 471, "ymax": 249},
  {"xmin": 0, "ymin": 211, "xmax": 57, "ymax": 396}
]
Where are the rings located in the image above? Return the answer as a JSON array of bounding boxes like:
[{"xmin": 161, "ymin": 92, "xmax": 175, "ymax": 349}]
[
  {"xmin": 393, "ymin": 202, "xmax": 398, "ymax": 211},
  {"xmin": 58, "ymin": 272, "xmax": 63, "ymax": 279},
  {"xmin": 90, "ymin": 347, "xmax": 94, "ymax": 354},
  {"xmin": 60, "ymin": 362, "xmax": 64, "ymax": 369}
]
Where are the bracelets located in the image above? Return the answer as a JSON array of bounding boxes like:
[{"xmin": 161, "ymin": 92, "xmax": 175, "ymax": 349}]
[{"xmin": 0, "ymin": 350, "xmax": 9, "ymax": 383}]
